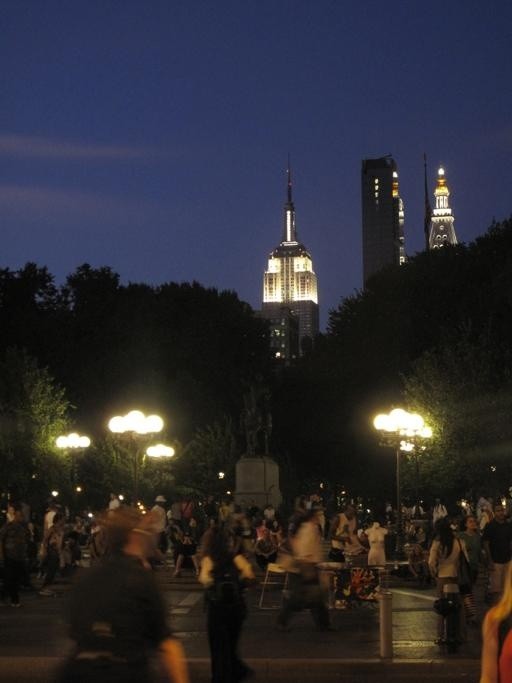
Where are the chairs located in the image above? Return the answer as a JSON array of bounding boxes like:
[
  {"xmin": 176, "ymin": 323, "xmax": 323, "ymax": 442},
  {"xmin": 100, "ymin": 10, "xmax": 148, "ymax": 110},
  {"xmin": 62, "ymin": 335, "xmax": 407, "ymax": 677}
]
[{"xmin": 255, "ymin": 561, "xmax": 289, "ymax": 611}]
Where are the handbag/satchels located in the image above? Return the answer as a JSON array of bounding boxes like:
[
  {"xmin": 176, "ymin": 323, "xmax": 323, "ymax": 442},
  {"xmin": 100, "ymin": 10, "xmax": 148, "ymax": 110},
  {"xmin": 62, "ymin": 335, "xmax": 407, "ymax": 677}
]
[{"xmin": 457, "ymin": 539, "xmax": 474, "ymax": 596}]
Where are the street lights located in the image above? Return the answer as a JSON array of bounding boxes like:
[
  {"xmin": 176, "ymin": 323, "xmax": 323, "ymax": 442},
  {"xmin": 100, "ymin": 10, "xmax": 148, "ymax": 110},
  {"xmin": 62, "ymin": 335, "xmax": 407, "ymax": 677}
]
[
  {"xmin": 374, "ymin": 408, "xmax": 434, "ymax": 552},
  {"xmin": 56, "ymin": 432, "xmax": 91, "ymax": 524},
  {"xmin": 107, "ymin": 412, "xmax": 175, "ymax": 503}
]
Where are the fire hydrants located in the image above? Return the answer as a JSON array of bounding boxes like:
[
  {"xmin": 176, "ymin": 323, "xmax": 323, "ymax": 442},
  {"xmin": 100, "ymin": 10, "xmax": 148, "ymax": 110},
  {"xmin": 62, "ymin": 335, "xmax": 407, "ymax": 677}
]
[{"xmin": 433, "ymin": 583, "xmax": 463, "ymax": 652}]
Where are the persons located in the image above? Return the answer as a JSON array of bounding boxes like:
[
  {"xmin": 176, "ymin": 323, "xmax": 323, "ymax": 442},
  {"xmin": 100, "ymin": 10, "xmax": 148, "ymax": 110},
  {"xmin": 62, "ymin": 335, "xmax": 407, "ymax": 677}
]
[
  {"xmin": 476, "ymin": 560, "xmax": 512, "ymax": 683},
  {"xmin": 190, "ymin": 522, "xmax": 257, "ymax": 682},
  {"xmin": 56, "ymin": 507, "xmax": 193, "ymax": 681}
]
